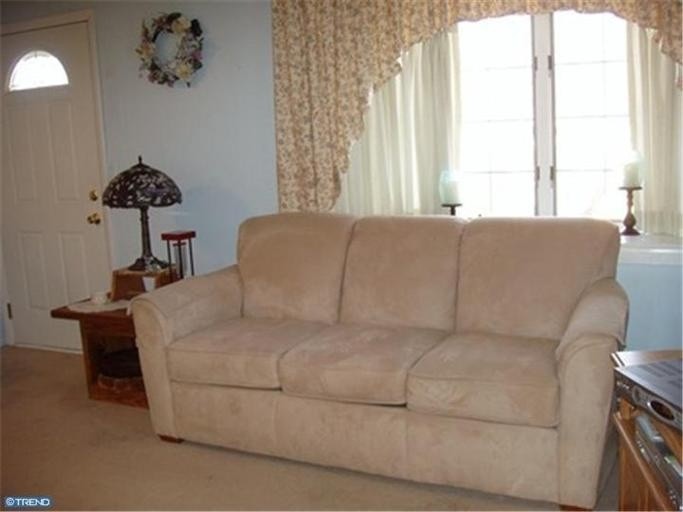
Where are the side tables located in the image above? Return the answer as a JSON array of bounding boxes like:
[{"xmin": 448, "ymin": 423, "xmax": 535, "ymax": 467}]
[{"xmin": 50, "ymin": 293, "xmax": 152, "ymax": 410}]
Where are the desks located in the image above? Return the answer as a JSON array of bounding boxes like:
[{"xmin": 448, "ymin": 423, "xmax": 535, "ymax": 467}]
[{"xmin": 609, "ymin": 348, "xmax": 682, "ymax": 511}]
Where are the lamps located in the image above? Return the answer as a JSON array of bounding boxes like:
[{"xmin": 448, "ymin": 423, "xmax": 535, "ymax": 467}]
[{"xmin": 101, "ymin": 153, "xmax": 181, "ymax": 301}]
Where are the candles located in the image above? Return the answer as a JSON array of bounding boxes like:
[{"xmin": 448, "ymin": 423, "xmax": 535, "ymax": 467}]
[
  {"xmin": 624, "ymin": 163, "xmax": 637, "ymax": 186},
  {"xmin": 443, "ymin": 183, "xmax": 458, "ymax": 205}
]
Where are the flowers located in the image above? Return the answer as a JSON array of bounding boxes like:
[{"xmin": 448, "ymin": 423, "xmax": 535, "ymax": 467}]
[{"xmin": 134, "ymin": 10, "xmax": 205, "ymax": 87}]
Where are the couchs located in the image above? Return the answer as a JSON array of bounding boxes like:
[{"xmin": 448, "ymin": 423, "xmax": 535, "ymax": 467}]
[{"xmin": 127, "ymin": 215, "xmax": 629, "ymax": 511}]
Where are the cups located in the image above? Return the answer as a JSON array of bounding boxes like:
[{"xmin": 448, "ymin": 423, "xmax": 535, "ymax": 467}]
[{"xmin": 89, "ymin": 293, "xmax": 107, "ymax": 305}]
